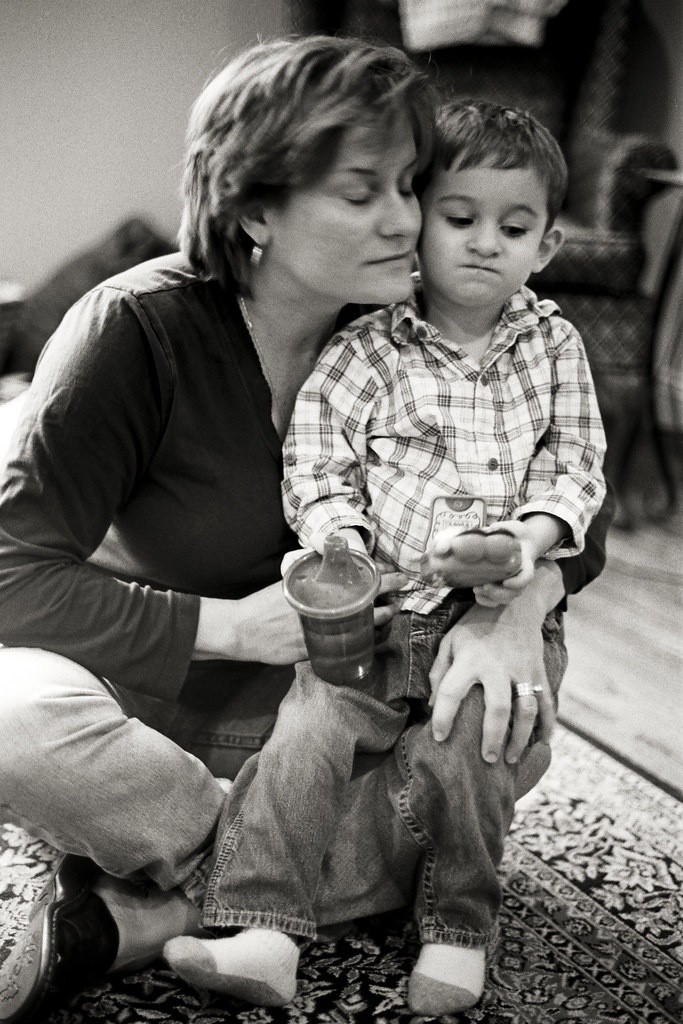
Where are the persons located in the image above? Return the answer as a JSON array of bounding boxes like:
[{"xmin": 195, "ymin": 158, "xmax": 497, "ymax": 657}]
[{"xmin": 0, "ymin": 32, "xmax": 617, "ymax": 1024}]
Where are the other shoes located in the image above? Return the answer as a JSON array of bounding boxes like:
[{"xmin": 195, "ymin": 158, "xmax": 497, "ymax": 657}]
[{"xmin": 32, "ymin": 855, "xmax": 122, "ymax": 1003}]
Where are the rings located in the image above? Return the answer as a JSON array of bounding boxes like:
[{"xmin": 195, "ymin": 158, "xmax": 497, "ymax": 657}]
[{"xmin": 514, "ymin": 681, "xmax": 542, "ymax": 698}]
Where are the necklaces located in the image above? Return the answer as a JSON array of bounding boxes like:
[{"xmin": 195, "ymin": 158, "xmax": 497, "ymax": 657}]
[{"xmin": 240, "ymin": 296, "xmax": 289, "ymax": 432}]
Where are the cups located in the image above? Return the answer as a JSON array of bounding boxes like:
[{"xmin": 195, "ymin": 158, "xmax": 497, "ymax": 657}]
[{"xmin": 282, "ymin": 537, "xmax": 382, "ymax": 685}]
